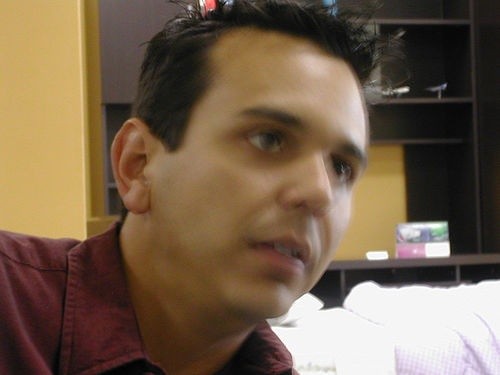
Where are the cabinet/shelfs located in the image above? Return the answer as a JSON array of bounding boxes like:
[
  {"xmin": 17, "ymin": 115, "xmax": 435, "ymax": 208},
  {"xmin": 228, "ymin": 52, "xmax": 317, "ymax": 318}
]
[
  {"xmin": 102, "ymin": 99, "xmax": 138, "ymax": 217},
  {"xmin": 307, "ymin": 0, "xmax": 500, "ymax": 311}
]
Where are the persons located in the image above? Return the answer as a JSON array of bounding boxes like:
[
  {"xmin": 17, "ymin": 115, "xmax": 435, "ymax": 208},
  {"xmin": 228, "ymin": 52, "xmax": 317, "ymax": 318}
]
[{"xmin": 0, "ymin": 0, "xmax": 409, "ymax": 375}]
